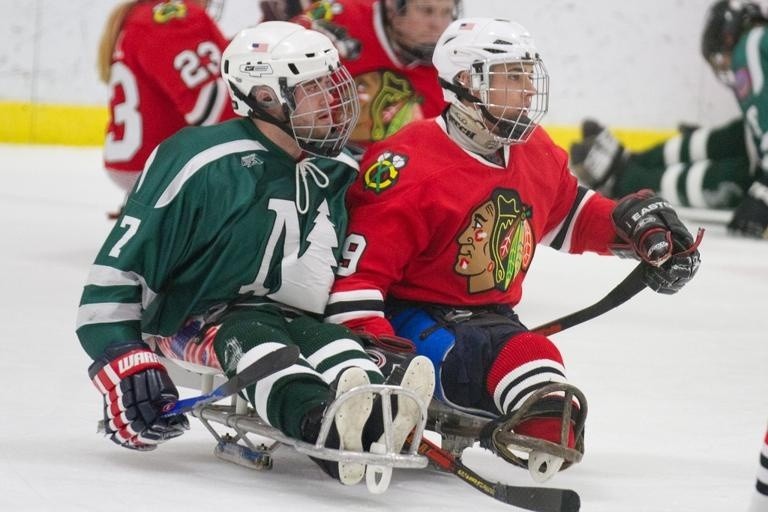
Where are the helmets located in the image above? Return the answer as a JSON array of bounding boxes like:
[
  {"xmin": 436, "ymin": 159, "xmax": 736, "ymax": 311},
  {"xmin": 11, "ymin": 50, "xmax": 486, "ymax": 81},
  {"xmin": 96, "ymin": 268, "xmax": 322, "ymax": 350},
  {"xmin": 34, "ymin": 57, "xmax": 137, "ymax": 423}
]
[
  {"xmin": 381, "ymin": 0, "xmax": 462, "ymax": 53},
  {"xmin": 699, "ymin": 1, "xmax": 762, "ymax": 65},
  {"xmin": 220, "ymin": 20, "xmax": 341, "ymax": 119},
  {"xmin": 432, "ymin": 16, "xmax": 540, "ymax": 104}
]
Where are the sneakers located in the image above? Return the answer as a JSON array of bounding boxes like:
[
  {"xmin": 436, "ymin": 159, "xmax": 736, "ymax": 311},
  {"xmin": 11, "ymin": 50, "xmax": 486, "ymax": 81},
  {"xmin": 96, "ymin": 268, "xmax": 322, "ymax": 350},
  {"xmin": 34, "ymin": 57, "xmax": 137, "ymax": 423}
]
[
  {"xmin": 570, "ymin": 121, "xmax": 634, "ymax": 200},
  {"xmin": 301, "ymin": 365, "xmax": 374, "ymax": 486},
  {"xmin": 362, "ymin": 354, "xmax": 437, "ymax": 475}
]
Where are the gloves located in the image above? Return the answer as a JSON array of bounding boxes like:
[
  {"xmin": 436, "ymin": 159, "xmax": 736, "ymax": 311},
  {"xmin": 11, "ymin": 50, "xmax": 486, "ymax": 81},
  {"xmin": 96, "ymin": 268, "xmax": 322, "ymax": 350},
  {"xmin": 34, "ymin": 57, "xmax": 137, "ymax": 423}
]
[
  {"xmin": 87, "ymin": 339, "xmax": 191, "ymax": 452},
  {"xmin": 606, "ymin": 187, "xmax": 702, "ymax": 295},
  {"xmin": 726, "ymin": 167, "xmax": 768, "ymax": 237}
]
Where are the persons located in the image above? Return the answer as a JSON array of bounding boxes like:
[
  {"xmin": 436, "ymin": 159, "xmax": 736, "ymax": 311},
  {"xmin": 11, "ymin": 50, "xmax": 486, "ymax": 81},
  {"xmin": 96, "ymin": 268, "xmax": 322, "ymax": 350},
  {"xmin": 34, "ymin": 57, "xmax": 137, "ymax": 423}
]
[
  {"xmin": 569, "ymin": 0, "xmax": 768, "ymax": 244},
  {"xmin": 453, "ymin": 188, "xmax": 536, "ymax": 295},
  {"xmin": 257, "ymin": 1, "xmax": 462, "ymax": 152},
  {"xmin": 97, "ymin": 2, "xmax": 243, "ymax": 194},
  {"xmin": 73, "ymin": 21, "xmax": 439, "ymax": 489},
  {"xmin": 322, "ymin": 14, "xmax": 703, "ymax": 486}
]
